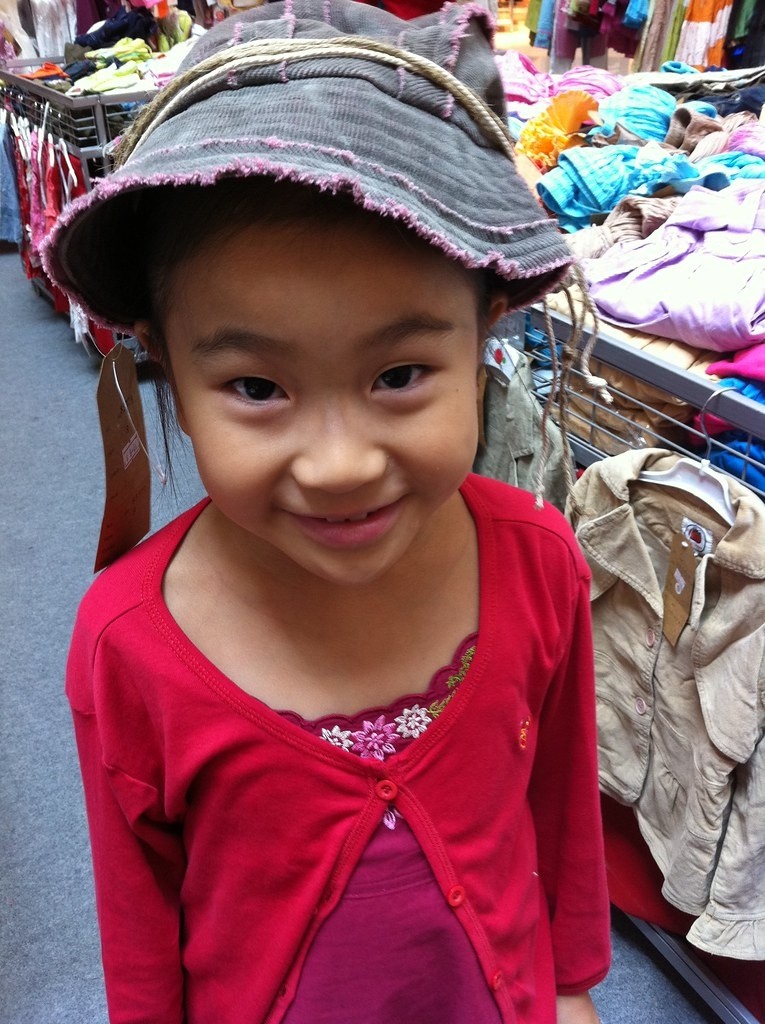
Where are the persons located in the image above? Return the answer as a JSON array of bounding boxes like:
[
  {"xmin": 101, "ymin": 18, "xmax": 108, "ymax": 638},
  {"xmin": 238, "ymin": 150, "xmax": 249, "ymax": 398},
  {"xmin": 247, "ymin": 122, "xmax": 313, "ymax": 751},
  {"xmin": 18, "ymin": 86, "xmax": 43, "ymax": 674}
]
[{"xmin": 36, "ymin": 1, "xmax": 613, "ymax": 1024}]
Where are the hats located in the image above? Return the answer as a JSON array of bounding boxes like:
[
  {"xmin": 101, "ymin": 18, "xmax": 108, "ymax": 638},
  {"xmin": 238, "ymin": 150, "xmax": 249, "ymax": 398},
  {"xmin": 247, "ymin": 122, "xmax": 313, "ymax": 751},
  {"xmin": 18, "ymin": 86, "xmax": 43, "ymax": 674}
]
[{"xmin": 40, "ymin": 0, "xmax": 573, "ymax": 335}]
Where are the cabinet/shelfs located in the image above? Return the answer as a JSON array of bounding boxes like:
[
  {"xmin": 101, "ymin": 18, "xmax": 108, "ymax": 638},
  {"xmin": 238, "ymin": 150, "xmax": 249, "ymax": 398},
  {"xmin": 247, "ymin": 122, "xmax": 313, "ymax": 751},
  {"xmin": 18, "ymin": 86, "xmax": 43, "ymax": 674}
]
[
  {"xmin": 0, "ymin": 53, "xmax": 161, "ymax": 369},
  {"xmin": 519, "ymin": 301, "xmax": 765, "ymax": 508}
]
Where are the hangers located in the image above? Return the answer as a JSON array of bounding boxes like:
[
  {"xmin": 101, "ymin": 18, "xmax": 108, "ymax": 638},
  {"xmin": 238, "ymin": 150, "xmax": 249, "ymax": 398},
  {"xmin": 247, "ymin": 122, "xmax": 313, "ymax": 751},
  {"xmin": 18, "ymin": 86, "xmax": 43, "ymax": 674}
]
[
  {"xmin": 5, "ymin": 92, "xmax": 81, "ymax": 190},
  {"xmin": 482, "ymin": 314, "xmax": 522, "ymax": 378},
  {"xmin": 631, "ymin": 388, "xmax": 744, "ymax": 527}
]
